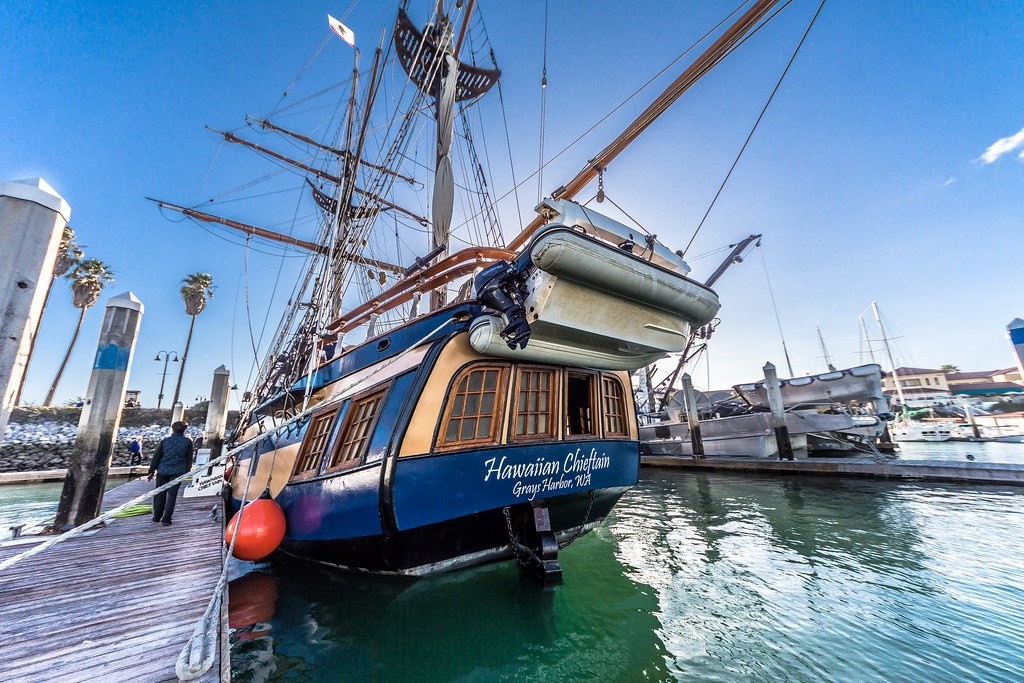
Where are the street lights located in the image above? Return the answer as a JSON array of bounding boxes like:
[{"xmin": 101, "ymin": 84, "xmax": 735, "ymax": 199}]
[
  {"xmin": 154, "ymin": 350, "xmax": 180, "ymax": 409},
  {"xmin": 194, "ymin": 394, "xmax": 207, "ymax": 403}
]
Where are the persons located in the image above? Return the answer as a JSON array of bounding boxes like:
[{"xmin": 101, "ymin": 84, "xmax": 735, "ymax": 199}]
[
  {"xmin": 148, "ymin": 422, "xmax": 193, "ymax": 526},
  {"xmin": 130, "ymin": 435, "xmax": 142, "ymax": 466},
  {"xmin": 186, "ymin": 431, "xmax": 204, "ymax": 465}
]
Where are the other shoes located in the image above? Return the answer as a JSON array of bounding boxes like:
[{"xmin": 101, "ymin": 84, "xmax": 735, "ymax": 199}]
[
  {"xmin": 162, "ymin": 519, "xmax": 172, "ymax": 524},
  {"xmin": 152, "ymin": 517, "xmax": 160, "ymax": 522}
]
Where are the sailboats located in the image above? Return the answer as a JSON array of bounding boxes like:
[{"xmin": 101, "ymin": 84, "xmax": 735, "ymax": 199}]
[
  {"xmin": 148, "ymin": 1, "xmax": 836, "ymax": 574},
  {"xmin": 853, "ymin": 300, "xmax": 1024, "ymax": 444}
]
